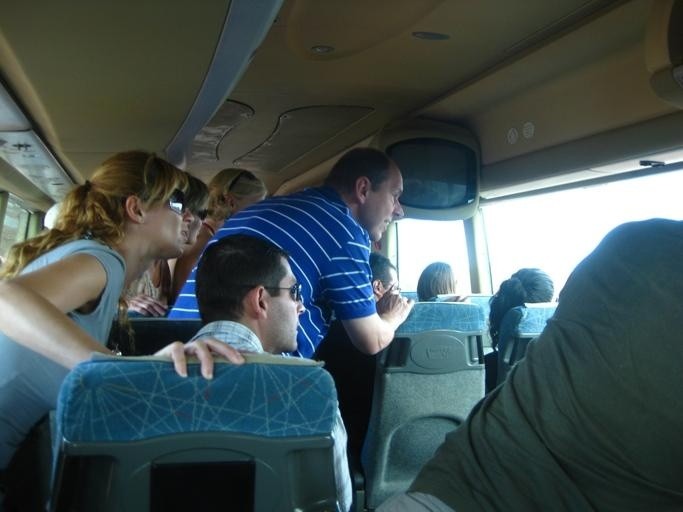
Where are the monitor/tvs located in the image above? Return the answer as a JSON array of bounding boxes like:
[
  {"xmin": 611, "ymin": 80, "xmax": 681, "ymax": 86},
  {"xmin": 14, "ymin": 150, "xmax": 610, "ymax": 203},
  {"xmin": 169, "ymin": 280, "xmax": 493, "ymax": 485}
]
[{"xmin": 367, "ymin": 118, "xmax": 482, "ymax": 220}]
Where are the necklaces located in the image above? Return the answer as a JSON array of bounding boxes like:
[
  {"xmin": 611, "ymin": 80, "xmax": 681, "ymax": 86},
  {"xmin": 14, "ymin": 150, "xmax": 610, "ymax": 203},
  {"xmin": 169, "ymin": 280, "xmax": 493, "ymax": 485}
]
[{"xmin": 202, "ymin": 222, "xmax": 216, "ymax": 236}]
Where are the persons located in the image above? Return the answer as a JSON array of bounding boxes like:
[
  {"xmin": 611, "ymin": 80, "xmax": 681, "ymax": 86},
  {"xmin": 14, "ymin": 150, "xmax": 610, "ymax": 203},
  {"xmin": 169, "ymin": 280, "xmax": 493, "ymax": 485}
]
[
  {"xmin": 369, "ymin": 215, "xmax": 682, "ymax": 511},
  {"xmin": 0, "ymin": 147, "xmax": 246, "ymax": 485},
  {"xmin": 483, "ymin": 266, "xmax": 556, "ymax": 396},
  {"xmin": 168, "ymin": 166, "xmax": 268, "ymax": 307},
  {"xmin": 182, "ymin": 232, "xmax": 353, "ymax": 511},
  {"xmin": 416, "ymin": 261, "xmax": 456, "ymax": 301},
  {"xmin": 367, "ymin": 252, "xmax": 397, "ymax": 302},
  {"xmin": 162, "ymin": 146, "xmax": 414, "ymax": 363},
  {"xmin": 126, "ymin": 172, "xmax": 210, "ymax": 317}
]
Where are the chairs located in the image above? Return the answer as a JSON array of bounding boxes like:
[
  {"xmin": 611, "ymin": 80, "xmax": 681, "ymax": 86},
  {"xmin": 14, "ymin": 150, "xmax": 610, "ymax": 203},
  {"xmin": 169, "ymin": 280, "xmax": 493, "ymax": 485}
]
[
  {"xmin": 361, "ymin": 302, "xmax": 486, "ymax": 511},
  {"xmin": 44, "ymin": 357, "xmax": 340, "ymax": 512},
  {"xmin": 120, "ymin": 316, "xmax": 203, "ymax": 357},
  {"xmin": 494, "ymin": 302, "xmax": 558, "ymax": 383},
  {"xmin": 460, "ymin": 293, "xmax": 495, "ymax": 357}
]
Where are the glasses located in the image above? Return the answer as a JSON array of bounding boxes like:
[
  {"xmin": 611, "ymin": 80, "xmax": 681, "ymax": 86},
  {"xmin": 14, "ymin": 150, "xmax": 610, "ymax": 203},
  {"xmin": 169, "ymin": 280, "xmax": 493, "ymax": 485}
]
[
  {"xmin": 150, "ymin": 187, "xmax": 188, "ymax": 214},
  {"xmin": 260, "ymin": 282, "xmax": 305, "ymax": 302}
]
